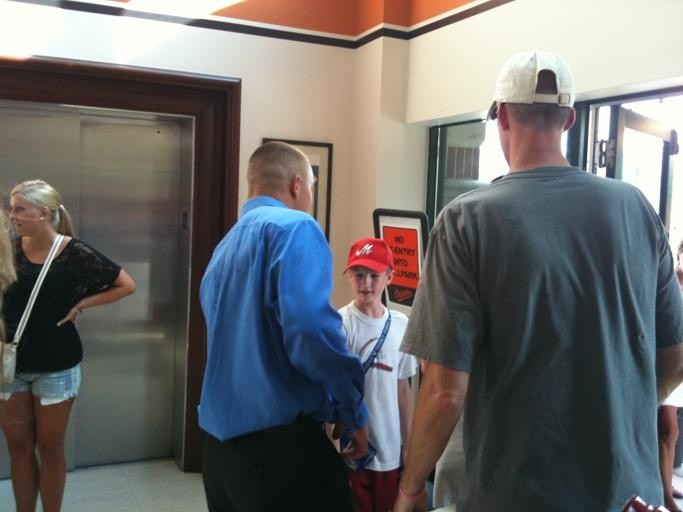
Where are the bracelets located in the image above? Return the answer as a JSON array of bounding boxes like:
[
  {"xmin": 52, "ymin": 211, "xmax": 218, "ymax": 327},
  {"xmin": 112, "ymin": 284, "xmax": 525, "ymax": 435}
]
[
  {"xmin": 70, "ymin": 306, "xmax": 82, "ymax": 315},
  {"xmin": 399, "ymin": 477, "xmax": 425, "ymax": 501}
]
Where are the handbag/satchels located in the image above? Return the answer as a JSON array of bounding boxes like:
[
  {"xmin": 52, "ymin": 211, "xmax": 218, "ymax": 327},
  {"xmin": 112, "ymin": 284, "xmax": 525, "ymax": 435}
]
[{"xmin": 1, "ymin": 338, "xmax": 19, "ymax": 383}]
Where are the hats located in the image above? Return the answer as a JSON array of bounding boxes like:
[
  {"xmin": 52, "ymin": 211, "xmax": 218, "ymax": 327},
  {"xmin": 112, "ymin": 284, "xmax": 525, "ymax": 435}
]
[
  {"xmin": 343, "ymin": 237, "xmax": 395, "ymax": 275},
  {"xmin": 485, "ymin": 50, "xmax": 577, "ymax": 122}
]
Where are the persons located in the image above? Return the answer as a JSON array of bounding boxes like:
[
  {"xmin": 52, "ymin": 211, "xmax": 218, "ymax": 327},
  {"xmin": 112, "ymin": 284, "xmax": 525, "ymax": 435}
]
[
  {"xmin": 198, "ymin": 139, "xmax": 368, "ymax": 511},
  {"xmin": 656, "ymin": 240, "xmax": 682, "ymax": 511},
  {"xmin": 331, "ymin": 237, "xmax": 420, "ymax": 512},
  {"xmin": 0, "ymin": 211, "xmax": 19, "ymax": 288},
  {"xmin": 392, "ymin": 46, "xmax": 683, "ymax": 512},
  {"xmin": 0, "ymin": 179, "xmax": 136, "ymax": 511}
]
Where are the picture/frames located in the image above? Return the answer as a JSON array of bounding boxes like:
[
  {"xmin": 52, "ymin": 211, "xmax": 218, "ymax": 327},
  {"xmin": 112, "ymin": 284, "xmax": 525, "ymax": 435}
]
[{"xmin": 262, "ymin": 137, "xmax": 332, "ymax": 245}]
